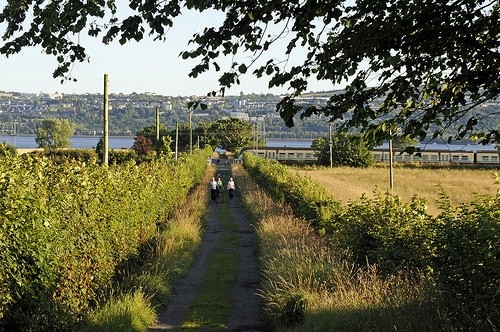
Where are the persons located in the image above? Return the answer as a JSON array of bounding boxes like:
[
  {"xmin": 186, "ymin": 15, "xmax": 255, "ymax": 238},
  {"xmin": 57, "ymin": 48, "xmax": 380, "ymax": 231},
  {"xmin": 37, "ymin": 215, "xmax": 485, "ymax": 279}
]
[
  {"xmin": 210, "ymin": 177, "xmax": 220, "ymax": 203},
  {"xmin": 217, "ymin": 177, "xmax": 223, "ymax": 198},
  {"xmin": 227, "ymin": 178, "xmax": 235, "ymax": 200}
]
